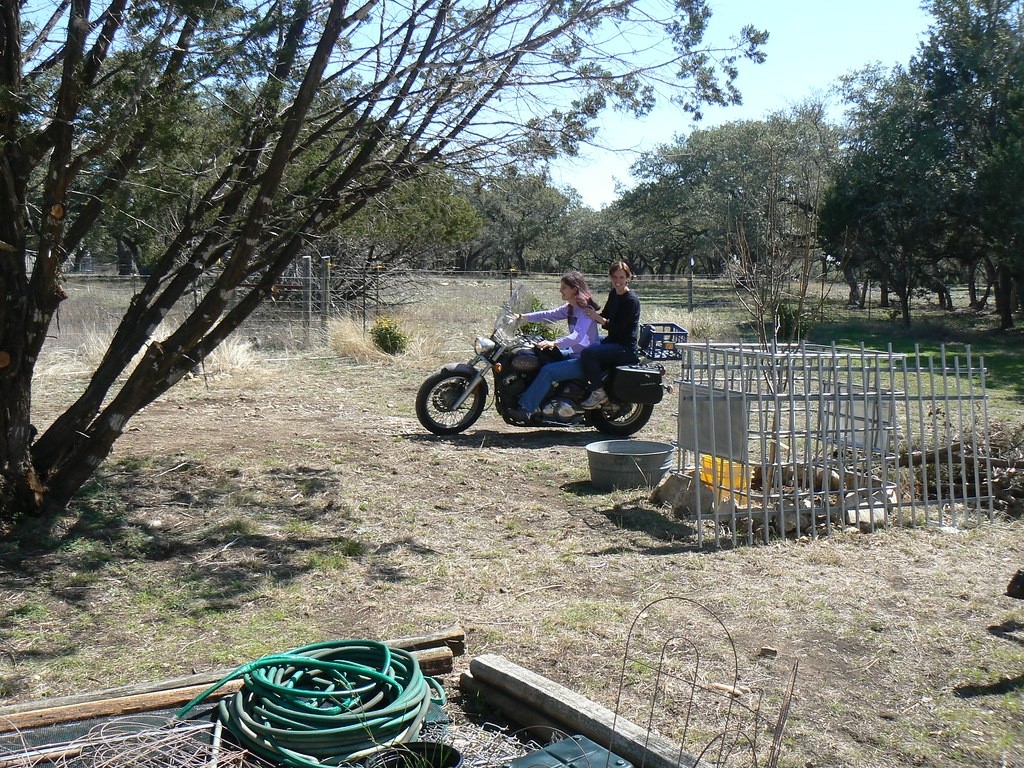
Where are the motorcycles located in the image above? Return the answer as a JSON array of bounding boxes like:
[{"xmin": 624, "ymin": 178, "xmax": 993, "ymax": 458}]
[{"xmin": 415, "ymin": 282, "xmax": 674, "ymax": 436}]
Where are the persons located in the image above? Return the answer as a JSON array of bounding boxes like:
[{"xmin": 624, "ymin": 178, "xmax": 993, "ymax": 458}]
[
  {"xmin": 581, "ymin": 262, "xmax": 641, "ymax": 409},
  {"xmin": 508, "ymin": 271, "xmax": 600, "ymax": 423}
]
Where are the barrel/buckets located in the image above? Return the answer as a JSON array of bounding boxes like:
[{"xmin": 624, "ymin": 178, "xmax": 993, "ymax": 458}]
[{"xmin": 702, "ymin": 457, "xmax": 752, "ymax": 507}]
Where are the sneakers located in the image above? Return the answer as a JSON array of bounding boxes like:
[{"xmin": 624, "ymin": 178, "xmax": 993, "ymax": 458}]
[
  {"xmin": 580, "ymin": 391, "xmax": 609, "ymax": 409},
  {"xmin": 508, "ymin": 408, "xmax": 530, "ymax": 424}
]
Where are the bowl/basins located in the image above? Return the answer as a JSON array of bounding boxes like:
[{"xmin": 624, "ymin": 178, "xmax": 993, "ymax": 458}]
[{"xmin": 585, "ymin": 440, "xmax": 676, "ymax": 492}]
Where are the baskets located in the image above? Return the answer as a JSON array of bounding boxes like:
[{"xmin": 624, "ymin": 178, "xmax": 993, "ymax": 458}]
[{"xmin": 639, "ymin": 322, "xmax": 689, "ymax": 360}]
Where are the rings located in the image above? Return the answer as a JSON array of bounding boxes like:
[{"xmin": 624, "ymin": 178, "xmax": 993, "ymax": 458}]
[{"xmin": 587, "ymin": 312, "xmax": 590, "ymax": 315}]
